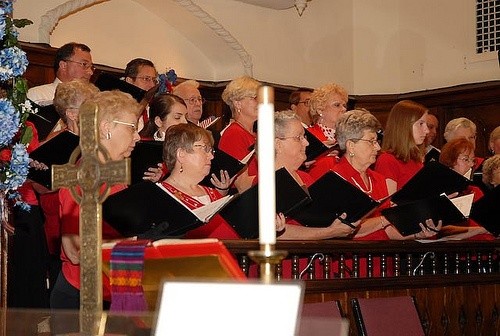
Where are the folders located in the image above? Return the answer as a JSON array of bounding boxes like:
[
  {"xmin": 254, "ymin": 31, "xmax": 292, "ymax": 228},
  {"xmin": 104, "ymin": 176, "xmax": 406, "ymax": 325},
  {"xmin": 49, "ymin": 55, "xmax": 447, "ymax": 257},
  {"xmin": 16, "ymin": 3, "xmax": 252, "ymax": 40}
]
[
  {"xmin": 471, "ymin": 156, "xmax": 488, "ymax": 186},
  {"xmin": 95, "ymin": 71, "xmax": 161, "ymax": 120},
  {"xmin": 25, "ymin": 96, "xmax": 62, "ymax": 141},
  {"xmin": 381, "ymin": 192, "xmax": 475, "ymax": 237},
  {"xmin": 219, "ymin": 167, "xmax": 312, "ymax": 240},
  {"xmin": 470, "ymin": 184, "xmax": 500, "ymax": 237},
  {"xmin": 130, "ymin": 140, "xmax": 164, "ymax": 177},
  {"xmin": 28, "ymin": 129, "xmax": 82, "ymax": 189},
  {"xmin": 297, "ymin": 127, "xmax": 383, "ymax": 162},
  {"xmin": 292, "ymin": 169, "xmax": 395, "ymax": 227},
  {"xmin": 391, "ymin": 158, "xmax": 473, "ymax": 207},
  {"xmin": 424, "ymin": 145, "xmax": 441, "ymax": 162},
  {"xmin": 201, "ymin": 114, "xmax": 256, "ymax": 189},
  {"xmin": 102, "ymin": 179, "xmax": 239, "ymax": 238}
]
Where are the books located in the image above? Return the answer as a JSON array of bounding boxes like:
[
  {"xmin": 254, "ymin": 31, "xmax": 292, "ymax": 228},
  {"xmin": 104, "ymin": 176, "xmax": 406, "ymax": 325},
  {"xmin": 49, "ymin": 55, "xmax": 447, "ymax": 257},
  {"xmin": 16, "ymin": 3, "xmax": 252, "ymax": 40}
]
[
  {"xmin": 390, "ymin": 160, "xmax": 474, "ymax": 205},
  {"xmin": 423, "ymin": 147, "xmax": 441, "ymax": 166},
  {"xmin": 26, "ymin": 98, "xmax": 61, "ymax": 142},
  {"xmin": 381, "ymin": 192, "xmax": 475, "ymax": 236},
  {"xmin": 102, "ymin": 180, "xmax": 232, "ymax": 239},
  {"xmin": 198, "ymin": 146, "xmax": 255, "ymax": 188},
  {"xmin": 291, "ymin": 170, "xmax": 397, "ymax": 228},
  {"xmin": 219, "ymin": 166, "xmax": 310, "ymax": 241},
  {"xmin": 94, "ymin": 72, "xmax": 160, "ymax": 108},
  {"xmin": 188, "ymin": 117, "xmax": 223, "ymax": 142},
  {"xmin": 131, "ymin": 139, "xmax": 163, "ymax": 182},
  {"xmin": 26, "ymin": 129, "xmax": 82, "ymax": 189},
  {"xmin": 298, "ymin": 126, "xmax": 339, "ymax": 172}
]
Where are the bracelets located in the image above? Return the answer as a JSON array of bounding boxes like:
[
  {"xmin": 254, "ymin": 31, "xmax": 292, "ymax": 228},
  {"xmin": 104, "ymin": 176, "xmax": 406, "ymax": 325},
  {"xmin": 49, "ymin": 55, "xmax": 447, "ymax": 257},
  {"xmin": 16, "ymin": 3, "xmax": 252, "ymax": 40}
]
[
  {"xmin": 414, "ymin": 234, "xmax": 420, "ymax": 239},
  {"xmin": 216, "ymin": 186, "xmax": 228, "ymax": 191},
  {"xmin": 276, "ymin": 228, "xmax": 286, "ymax": 238}
]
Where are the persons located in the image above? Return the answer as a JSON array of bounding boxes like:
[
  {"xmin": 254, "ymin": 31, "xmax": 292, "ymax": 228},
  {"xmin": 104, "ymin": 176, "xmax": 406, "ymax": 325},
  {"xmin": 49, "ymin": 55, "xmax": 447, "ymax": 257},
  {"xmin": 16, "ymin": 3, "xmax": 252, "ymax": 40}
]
[
  {"xmin": 219, "ymin": 74, "xmax": 500, "ymax": 280},
  {"xmin": 0, "ymin": 41, "xmax": 236, "ymax": 310}
]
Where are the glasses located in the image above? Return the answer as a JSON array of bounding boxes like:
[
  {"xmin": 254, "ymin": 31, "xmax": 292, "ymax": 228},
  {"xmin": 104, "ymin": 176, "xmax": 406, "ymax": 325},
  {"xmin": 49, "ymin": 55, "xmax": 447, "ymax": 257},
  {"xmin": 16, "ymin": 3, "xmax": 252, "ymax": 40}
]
[
  {"xmin": 458, "ymin": 156, "xmax": 478, "ymax": 165},
  {"xmin": 184, "ymin": 97, "xmax": 202, "ymax": 104},
  {"xmin": 281, "ymin": 135, "xmax": 307, "ymax": 142},
  {"xmin": 468, "ymin": 136, "xmax": 476, "ymax": 143},
  {"xmin": 298, "ymin": 99, "xmax": 310, "ymax": 107},
  {"xmin": 64, "ymin": 58, "xmax": 96, "ymax": 72},
  {"xmin": 136, "ymin": 76, "xmax": 159, "ymax": 85},
  {"xmin": 358, "ymin": 139, "xmax": 381, "ymax": 146},
  {"xmin": 193, "ymin": 144, "xmax": 215, "ymax": 156}
]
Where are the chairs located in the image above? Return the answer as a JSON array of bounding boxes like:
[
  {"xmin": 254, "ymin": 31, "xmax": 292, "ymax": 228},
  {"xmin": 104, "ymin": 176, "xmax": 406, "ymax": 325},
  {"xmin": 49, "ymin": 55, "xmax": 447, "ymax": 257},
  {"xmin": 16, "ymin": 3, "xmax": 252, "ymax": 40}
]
[
  {"xmin": 302, "ymin": 301, "xmax": 350, "ymax": 336},
  {"xmin": 351, "ymin": 296, "xmax": 428, "ymax": 336}
]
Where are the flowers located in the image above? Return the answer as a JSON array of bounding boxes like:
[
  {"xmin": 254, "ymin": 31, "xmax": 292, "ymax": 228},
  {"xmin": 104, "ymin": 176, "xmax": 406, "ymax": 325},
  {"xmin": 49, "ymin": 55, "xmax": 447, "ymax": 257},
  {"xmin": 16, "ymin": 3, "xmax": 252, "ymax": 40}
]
[{"xmin": 0, "ymin": 0, "xmax": 48, "ymax": 212}]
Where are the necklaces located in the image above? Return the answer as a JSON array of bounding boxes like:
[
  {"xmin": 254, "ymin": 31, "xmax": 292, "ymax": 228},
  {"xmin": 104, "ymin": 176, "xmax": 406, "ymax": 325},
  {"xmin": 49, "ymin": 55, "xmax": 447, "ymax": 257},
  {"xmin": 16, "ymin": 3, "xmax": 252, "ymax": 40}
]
[{"xmin": 352, "ymin": 176, "xmax": 372, "ymax": 193}]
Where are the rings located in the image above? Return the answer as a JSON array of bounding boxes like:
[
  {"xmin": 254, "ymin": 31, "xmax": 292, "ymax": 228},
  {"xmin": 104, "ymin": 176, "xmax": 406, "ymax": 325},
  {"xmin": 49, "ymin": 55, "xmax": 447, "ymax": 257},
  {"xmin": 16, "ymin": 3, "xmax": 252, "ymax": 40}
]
[{"xmin": 222, "ymin": 182, "xmax": 226, "ymax": 184}]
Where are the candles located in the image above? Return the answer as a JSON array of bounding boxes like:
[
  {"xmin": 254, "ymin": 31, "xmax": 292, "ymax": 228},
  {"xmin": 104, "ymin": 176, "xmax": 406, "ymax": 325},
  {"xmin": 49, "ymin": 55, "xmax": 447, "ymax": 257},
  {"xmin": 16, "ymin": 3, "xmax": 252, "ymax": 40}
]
[{"xmin": 258, "ymin": 86, "xmax": 277, "ymax": 244}]
